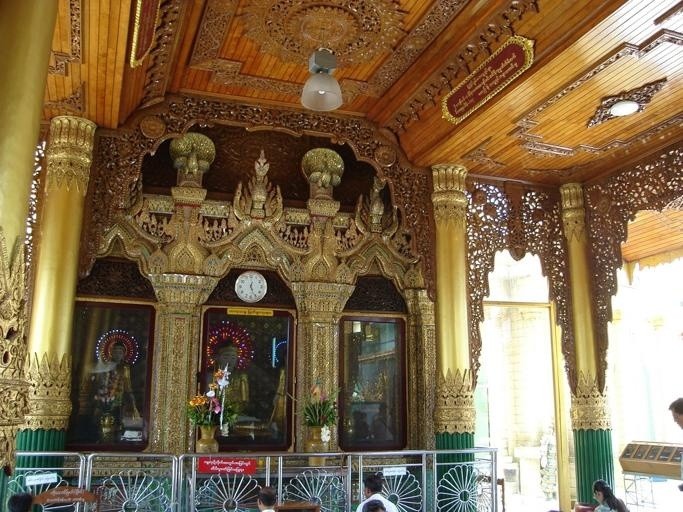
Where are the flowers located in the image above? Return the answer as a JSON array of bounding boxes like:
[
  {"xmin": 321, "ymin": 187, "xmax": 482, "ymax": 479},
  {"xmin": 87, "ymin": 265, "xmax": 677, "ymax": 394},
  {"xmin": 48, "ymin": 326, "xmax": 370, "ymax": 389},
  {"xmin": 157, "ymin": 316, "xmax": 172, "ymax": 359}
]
[
  {"xmin": 283, "ymin": 378, "xmax": 344, "ymax": 445},
  {"xmin": 180, "ymin": 362, "xmax": 243, "ymax": 432},
  {"xmin": 91, "ymin": 386, "xmax": 124, "ymax": 413}
]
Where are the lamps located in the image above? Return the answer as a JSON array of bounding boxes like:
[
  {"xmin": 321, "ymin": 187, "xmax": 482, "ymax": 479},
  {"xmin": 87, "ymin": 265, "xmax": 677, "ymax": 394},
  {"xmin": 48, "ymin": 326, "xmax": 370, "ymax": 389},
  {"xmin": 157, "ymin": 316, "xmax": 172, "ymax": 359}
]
[
  {"xmin": 605, "ymin": 99, "xmax": 640, "ymax": 118},
  {"xmin": 299, "ymin": 44, "xmax": 344, "ymax": 113}
]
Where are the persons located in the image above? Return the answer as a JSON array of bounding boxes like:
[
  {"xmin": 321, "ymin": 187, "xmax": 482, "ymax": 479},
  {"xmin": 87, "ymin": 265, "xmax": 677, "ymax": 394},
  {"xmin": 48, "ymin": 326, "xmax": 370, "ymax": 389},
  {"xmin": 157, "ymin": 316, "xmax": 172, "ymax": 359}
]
[
  {"xmin": 7, "ymin": 493, "xmax": 32, "ymax": 512},
  {"xmin": 668, "ymin": 398, "xmax": 683, "ymax": 491},
  {"xmin": 257, "ymin": 487, "xmax": 276, "ymax": 512},
  {"xmin": 361, "ymin": 500, "xmax": 386, "ymax": 512},
  {"xmin": 356, "ymin": 475, "xmax": 398, "ymax": 512},
  {"xmin": 94, "ymin": 339, "xmax": 139, "ymax": 440},
  {"xmin": 592, "ymin": 478, "xmax": 629, "ymax": 512},
  {"xmin": 216, "ymin": 341, "xmax": 249, "ymax": 427}
]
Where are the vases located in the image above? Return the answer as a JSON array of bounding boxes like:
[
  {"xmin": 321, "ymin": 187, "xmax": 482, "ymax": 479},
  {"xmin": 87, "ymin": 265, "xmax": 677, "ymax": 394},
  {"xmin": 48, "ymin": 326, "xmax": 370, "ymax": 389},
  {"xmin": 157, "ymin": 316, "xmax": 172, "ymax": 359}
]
[
  {"xmin": 96, "ymin": 410, "xmax": 118, "ymax": 444},
  {"xmin": 303, "ymin": 425, "xmax": 330, "ymax": 467},
  {"xmin": 191, "ymin": 423, "xmax": 220, "ymax": 454}
]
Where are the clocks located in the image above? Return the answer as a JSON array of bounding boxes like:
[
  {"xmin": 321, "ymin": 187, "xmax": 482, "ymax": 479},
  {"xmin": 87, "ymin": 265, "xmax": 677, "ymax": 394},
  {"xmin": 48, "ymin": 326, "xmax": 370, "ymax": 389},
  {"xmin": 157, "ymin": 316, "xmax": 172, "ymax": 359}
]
[{"xmin": 231, "ymin": 268, "xmax": 268, "ymax": 305}]
